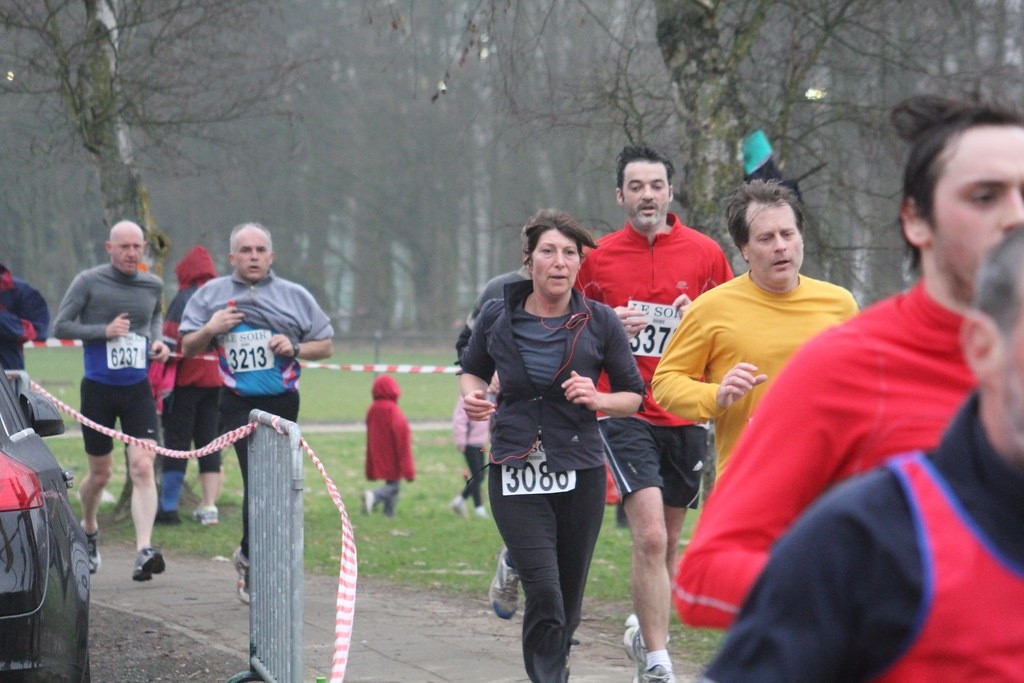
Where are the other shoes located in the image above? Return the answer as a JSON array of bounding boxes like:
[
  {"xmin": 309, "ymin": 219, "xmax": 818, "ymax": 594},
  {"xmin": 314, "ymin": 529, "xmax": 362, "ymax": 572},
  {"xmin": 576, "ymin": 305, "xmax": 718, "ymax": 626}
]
[
  {"xmin": 454, "ymin": 496, "xmax": 468, "ymax": 518},
  {"xmin": 191, "ymin": 501, "xmax": 220, "ymax": 525},
  {"xmin": 361, "ymin": 489, "xmax": 376, "ymax": 516},
  {"xmin": 155, "ymin": 502, "xmax": 183, "ymax": 527},
  {"xmin": 475, "ymin": 506, "xmax": 488, "ymax": 519}
]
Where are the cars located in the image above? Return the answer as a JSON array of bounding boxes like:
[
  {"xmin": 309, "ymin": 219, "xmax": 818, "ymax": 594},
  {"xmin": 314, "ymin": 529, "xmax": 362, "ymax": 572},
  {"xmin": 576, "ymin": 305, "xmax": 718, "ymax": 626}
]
[{"xmin": 0, "ymin": 368, "xmax": 93, "ymax": 683}]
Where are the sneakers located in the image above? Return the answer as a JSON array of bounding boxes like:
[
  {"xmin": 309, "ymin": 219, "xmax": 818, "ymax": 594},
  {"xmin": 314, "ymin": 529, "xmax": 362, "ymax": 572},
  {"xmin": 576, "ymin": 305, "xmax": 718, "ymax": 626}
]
[
  {"xmin": 83, "ymin": 528, "xmax": 101, "ymax": 574},
  {"xmin": 488, "ymin": 547, "xmax": 520, "ymax": 620},
  {"xmin": 232, "ymin": 546, "xmax": 256, "ymax": 605},
  {"xmin": 133, "ymin": 548, "xmax": 166, "ymax": 582},
  {"xmin": 623, "ymin": 626, "xmax": 677, "ymax": 683}
]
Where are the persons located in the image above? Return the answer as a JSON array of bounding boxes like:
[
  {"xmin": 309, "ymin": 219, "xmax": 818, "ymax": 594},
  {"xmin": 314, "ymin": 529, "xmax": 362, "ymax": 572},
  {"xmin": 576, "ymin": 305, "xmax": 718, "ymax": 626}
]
[
  {"xmin": 178, "ymin": 222, "xmax": 334, "ymax": 605},
  {"xmin": 53, "ymin": 220, "xmax": 170, "ymax": 581},
  {"xmin": 651, "ymin": 178, "xmax": 859, "ymax": 484},
  {"xmin": 451, "ymin": 394, "xmax": 491, "ymax": 520},
  {"xmin": 460, "ymin": 213, "xmax": 648, "ymax": 683},
  {"xmin": 155, "ymin": 243, "xmax": 223, "ymax": 525},
  {"xmin": 454, "ymin": 227, "xmax": 580, "ymax": 644},
  {"xmin": 0, "ymin": 263, "xmax": 49, "ymax": 371},
  {"xmin": 671, "ymin": 98, "xmax": 1022, "ymax": 628},
  {"xmin": 361, "ymin": 374, "xmax": 416, "ymax": 517},
  {"xmin": 572, "ymin": 145, "xmax": 736, "ymax": 683},
  {"xmin": 698, "ymin": 223, "xmax": 1023, "ymax": 682}
]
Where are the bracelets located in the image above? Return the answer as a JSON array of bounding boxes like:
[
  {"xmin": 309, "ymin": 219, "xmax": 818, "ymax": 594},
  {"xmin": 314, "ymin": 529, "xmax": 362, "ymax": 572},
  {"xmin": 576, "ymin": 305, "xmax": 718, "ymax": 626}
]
[{"xmin": 292, "ymin": 342, "xmax": 300, "ymax": 358}]
